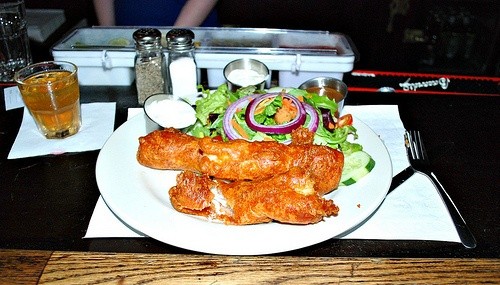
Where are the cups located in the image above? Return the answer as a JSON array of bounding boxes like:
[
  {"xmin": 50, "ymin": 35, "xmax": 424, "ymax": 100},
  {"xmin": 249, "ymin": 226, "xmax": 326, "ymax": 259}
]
[
  {"xmin": 14, "ymin": 61, "xmax": 82, "ymax": 139},
  {"xmin": 0, "ymin": 0, "xmax": 34, "ymax": 86}
]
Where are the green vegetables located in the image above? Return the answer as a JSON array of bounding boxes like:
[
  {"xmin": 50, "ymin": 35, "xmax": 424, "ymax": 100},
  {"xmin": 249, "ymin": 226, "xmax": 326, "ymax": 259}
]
[{"xmin": 189, "ymin": 86, "xmax": 362, "ymax": 156}]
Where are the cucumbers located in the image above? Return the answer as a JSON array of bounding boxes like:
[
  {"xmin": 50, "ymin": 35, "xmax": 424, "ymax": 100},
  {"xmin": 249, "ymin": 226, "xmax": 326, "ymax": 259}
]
[{"xmin": 341, "ymin": 150, "xmax": 375, "ymax": 187}]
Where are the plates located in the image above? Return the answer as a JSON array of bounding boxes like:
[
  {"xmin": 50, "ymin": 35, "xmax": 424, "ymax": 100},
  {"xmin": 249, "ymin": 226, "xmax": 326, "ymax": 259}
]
[{"xmin": 96, "ymin": 89, "xmax": 392, "ymax": 255}]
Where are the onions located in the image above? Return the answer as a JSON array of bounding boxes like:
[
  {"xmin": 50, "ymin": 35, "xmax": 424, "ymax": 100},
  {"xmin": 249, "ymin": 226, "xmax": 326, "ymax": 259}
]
[
  {"xmin": 282, "ymin": 102, "xmax": 318, "ymax": 145},
  {"xmin": 222, "ymin": 93, "xmax": 264, "ymax": 141},
  {"xmin": 245, "ymin": 91, "xmax": 307, "ymax": 132}
]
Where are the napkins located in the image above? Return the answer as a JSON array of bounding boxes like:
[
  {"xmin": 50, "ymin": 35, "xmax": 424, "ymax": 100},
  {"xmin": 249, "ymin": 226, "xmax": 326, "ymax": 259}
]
[
  {"xmin": 82, "ymin": 104, "xmax": 461, "ymax": 243},
  {"xmin": 8, "ymin": 101, "xmax": 116, "ymax": 159}
]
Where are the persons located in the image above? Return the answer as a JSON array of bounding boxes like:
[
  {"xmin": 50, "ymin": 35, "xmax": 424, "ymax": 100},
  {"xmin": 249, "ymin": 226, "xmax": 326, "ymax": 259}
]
[{"xmin": 92, "ymin": 0, "xmax": 218, "ymax": 27}]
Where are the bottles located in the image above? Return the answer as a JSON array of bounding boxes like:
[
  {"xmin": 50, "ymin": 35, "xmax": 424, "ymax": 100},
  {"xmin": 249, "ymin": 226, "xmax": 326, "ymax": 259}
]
[
  {"xmin": 133, "ymin": 29, "xmax": 164, "ymax": 104},
  {"xmin": 165, "ymin": 27, "xmax": 197, "ymax": 105}
]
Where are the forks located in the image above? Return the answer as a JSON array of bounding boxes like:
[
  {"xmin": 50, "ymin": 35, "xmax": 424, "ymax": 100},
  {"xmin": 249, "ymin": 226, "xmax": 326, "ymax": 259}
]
[{"xmin": 404, "ymin": 131, "xmax": 479, "ymax": 249}]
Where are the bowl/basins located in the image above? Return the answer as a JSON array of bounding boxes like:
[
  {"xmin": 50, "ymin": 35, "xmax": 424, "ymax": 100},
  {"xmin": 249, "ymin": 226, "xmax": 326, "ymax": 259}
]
[
  {"xmin": 144, "ymin": 94, "xmax": 198, "ymax": 137},
  {"xmin": 223, "ymin": 57, "xmax": 269, "ymax": 92},
  {"xmin": 299, "ymin": 78, "xmax": 346, "ymax": 114}
]
